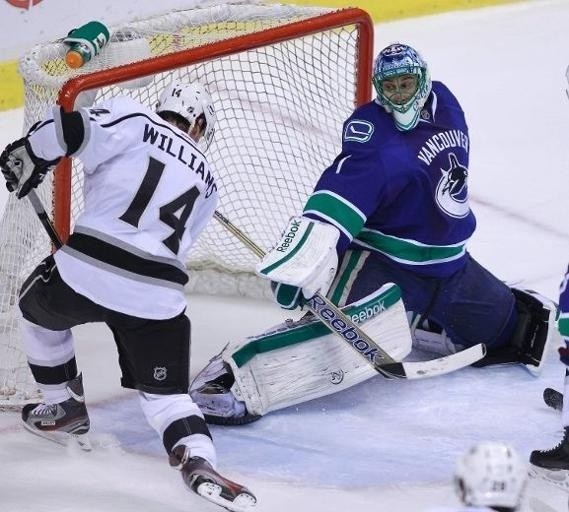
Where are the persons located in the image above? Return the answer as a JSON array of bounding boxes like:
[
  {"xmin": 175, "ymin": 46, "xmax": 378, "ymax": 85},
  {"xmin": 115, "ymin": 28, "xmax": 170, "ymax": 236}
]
[
  {"xmin": 181, "ymin": 41, "xmax": 520, "ymax": 427},
  {"xmin": 526, "ymin": 263, "xmax": 569, "ymax": 475},
  {"xmin": 1, "ymin": 77, "xmax": 261, "ymax": 509}
]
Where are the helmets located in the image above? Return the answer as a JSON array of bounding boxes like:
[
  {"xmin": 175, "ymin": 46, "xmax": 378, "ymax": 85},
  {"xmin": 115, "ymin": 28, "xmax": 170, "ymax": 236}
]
[
  {"xmin": 154, "ymin": 78, "xmax": 218, "ymax": 155},
  {"xmin": 370, "ymin": 42, "xmax": 433, "ymax": 132}
]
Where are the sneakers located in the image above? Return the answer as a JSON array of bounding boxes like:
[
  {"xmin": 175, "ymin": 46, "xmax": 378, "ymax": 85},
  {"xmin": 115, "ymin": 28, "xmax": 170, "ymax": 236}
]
[
  {"xmin": 20, "ymin": 396, "xmax": 92, "ymax": 435},
  {"xmin": 529, "ymin": 426, "xmax": 569, "ymax": 470},
  {"xmin": 181, "ymin": 456, "xmax": 257, "ymax": 511}
]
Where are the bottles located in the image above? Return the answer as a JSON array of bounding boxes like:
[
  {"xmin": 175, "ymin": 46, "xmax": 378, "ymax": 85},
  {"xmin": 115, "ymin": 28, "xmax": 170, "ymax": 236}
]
[{"xmin": 65, "ymin": 42, "xmax": 91, "ymax": 68}]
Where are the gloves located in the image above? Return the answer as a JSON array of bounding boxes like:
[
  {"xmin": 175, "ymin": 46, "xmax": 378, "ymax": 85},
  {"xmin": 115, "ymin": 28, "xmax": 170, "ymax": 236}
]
[
  {"xmin": 269, "ymin": 282, "xmax": 305, "ymax": 310},
  {"xmin": 0, "ymin": 134, "xmax": 62, "ymax": 200}
]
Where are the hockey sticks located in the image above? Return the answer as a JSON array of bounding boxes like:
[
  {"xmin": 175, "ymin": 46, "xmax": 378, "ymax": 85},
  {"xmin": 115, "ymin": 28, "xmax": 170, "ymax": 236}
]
[{"xmin": 213, "ymin": 210, "xmax": 487, "ymax": 380}]
